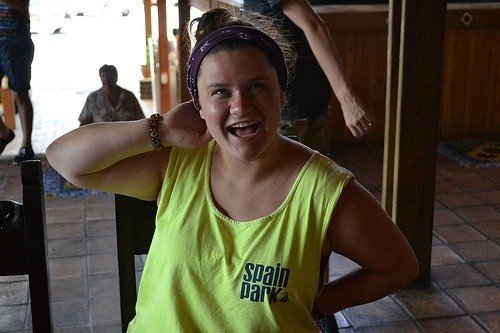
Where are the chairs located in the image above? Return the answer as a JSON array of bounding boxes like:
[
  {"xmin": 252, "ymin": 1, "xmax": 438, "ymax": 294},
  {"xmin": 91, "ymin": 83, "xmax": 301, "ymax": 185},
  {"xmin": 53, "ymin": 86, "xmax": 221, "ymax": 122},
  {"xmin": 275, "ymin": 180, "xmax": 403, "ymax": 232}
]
[
  {"xmin": 115, "ymin": 189, "xmax": 163, "ymax": 333},
  {"xmin": 0, "ymin": 161, "xmax": 53, "ymax": 333}
]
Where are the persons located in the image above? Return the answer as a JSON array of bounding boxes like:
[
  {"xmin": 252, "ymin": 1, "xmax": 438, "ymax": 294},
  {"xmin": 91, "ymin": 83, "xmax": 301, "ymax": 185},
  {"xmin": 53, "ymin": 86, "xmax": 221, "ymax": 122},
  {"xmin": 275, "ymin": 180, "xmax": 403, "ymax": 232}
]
[
  {"xmin": 243, "ymin": 0, "xmax": 376, "ymax": 160},
  {"xmin": 77, "ymin": 64, "xmax": 146, "ymax": 127},
  {"xmin": 45, "ymin": 8, "xmax": 418, "ymax": 333},
  {"xmin": 0, "ymin": 0, "xmax": 35, "ymax": 164}
]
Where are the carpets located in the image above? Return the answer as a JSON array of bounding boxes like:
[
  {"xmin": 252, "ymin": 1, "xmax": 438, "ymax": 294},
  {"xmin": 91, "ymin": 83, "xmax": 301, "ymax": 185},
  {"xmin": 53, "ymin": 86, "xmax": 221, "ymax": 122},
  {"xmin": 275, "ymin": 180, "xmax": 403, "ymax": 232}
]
[
  {"xmin": 440, "ymin": 139, "xmax": 500, "ymax": 170},
  {"xmin": 39, "ymin": 156, "xmax": 115, "ymax": 204}
]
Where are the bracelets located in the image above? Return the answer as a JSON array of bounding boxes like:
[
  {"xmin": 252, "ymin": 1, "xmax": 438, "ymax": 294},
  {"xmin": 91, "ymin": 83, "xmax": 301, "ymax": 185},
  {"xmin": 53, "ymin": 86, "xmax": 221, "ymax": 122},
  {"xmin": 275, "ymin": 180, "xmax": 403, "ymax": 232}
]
[{"xmin": 149, "ymin": 113, "xmax": 166, "ymax": 150}]
